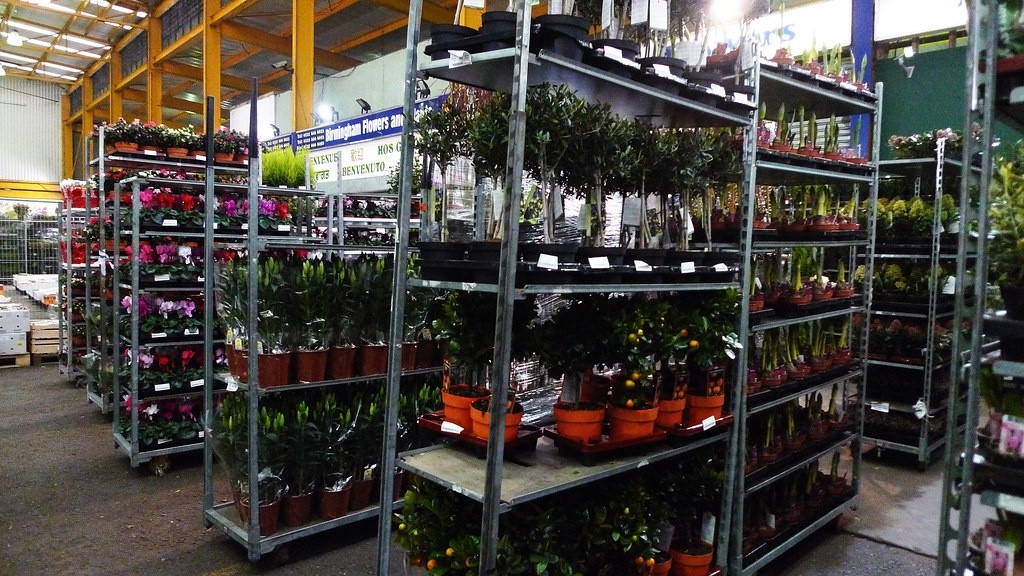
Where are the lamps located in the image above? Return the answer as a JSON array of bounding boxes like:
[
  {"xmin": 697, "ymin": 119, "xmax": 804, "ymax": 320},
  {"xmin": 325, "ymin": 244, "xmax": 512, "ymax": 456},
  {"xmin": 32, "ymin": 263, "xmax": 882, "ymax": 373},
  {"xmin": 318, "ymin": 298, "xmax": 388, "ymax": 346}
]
[{"xmin": 892, "ymin": 53, "xmax": 916, "ymax": 79}]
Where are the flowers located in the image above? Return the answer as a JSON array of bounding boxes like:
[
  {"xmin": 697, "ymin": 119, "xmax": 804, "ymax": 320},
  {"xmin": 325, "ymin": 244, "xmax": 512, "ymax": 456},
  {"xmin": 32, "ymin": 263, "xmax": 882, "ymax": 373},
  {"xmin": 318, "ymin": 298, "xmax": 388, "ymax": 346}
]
[
  {"xmin": 14, "ymin": 203, "xmax": 30, "ymax": 214},
  {"xmin": 60, "ymin": 118, "xmax": 293, "ymax": 439}
]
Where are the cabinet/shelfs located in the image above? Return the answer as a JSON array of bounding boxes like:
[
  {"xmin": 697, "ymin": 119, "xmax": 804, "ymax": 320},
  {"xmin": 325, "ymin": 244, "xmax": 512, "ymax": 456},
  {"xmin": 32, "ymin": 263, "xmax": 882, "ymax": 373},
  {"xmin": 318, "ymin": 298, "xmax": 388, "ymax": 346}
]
[
  {"xmin": 936, "ymin": 0, "xmax": 1024, "ymax": 576},
  {"xmin": 200, "ymin": 74, "xmax": 408, "ymax": 562},
  {"xmin": 820, "ymin": 135, "xmax": 995, "ymax": 469},
  {"xmin": 684, "ymin": 37, "xmax": 884, "ymax": 576},
  {"xmin": 79, "ymin": 122, "xmax": 248, "ymax": 412},
  {"xmin": 112, "ymin": 169, "xmax": 334, "ymax": 473},
  {"xmin": 372, "ymin": 4, "xmax": 757, "ymax": 576},
  {"xmin": 57, "ymin": 199, "xmax": 128, "ymax": 381},
  {"xmin": 315, "ymin": 191, "xmax": 442, "ymax": 231}
]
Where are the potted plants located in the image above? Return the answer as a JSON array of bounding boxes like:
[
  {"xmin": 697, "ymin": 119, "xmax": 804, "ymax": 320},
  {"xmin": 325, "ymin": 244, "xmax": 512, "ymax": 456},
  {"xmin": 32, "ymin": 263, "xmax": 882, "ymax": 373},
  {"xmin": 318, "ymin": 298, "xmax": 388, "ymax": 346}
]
[{"xmin": 205, "ymin": 0, "xmax": 1024, "ymax": 576}]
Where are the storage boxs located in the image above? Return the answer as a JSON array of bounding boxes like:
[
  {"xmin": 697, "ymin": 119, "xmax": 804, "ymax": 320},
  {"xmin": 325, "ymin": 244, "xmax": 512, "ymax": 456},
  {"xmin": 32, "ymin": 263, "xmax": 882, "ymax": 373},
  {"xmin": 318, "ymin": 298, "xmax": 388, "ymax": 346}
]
[
  {"xmin": 0, "ymin": 332, "xmax": 26, "ymax": 354},
  {"xmin": 0, "ymin": 304, "xmax": 31, "ymax": 334}
]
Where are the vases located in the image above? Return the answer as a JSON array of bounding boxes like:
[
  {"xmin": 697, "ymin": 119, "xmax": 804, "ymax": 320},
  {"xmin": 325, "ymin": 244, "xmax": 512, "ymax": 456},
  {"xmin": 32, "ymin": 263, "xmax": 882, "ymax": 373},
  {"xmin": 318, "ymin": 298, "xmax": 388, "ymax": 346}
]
[
  {"xmin": 236, "ymin": 155, "xmax": 249, "ymax": 164},
  {"xmin": 167, "ymin": 147, "xmax": 189, "ymax": 157},
  {"xmin": 73, "ymin": 337, "xmax": 85, "ymax": 346},
  {"xmin": 192, "ymin": 151, "xmax": 206, "ymax": 157},
  {"xmin": 64, "ymin": 312, "xmax": 83, "ymax": 323},
  {"xmin": 106, "ymin": 145, "xmax": 118, "ymax": 152},
  {"xmin": 17, "ymin": 214, "xmax": 25, "ymax": 220},
  {"xmin": 142, "ymin": 146, "xmax": 159, "ymax": 152},
  {"xmin": 115, "ymin": 142, "xmax": 139, "ymax": 152},
  {"xmin": 63, "ymin": 287, "xmax": 99, "ymax": 298},
  {"xmin": 92, "ymin": 241, "xmax": 127, "ymax": 249},
  {"xmin": 215, "ymin": 152, "xmax": 235, "ymax": 162}
]
